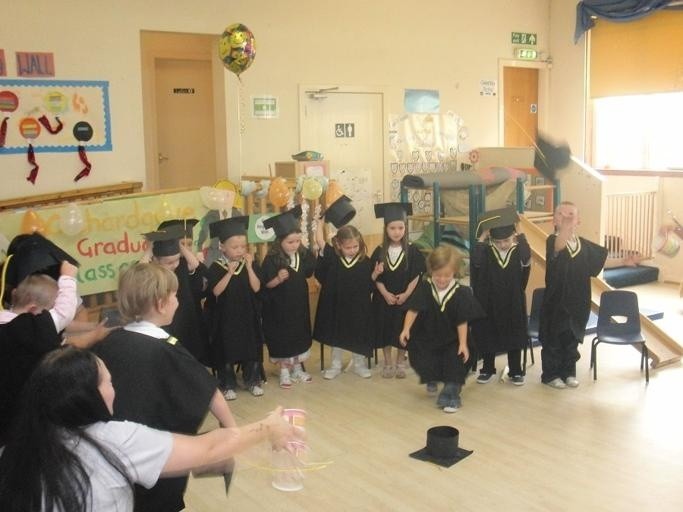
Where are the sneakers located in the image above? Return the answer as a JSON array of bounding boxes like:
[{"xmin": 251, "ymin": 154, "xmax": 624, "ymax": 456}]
[
  {"xmin": 476, "ymin": 370, "xmax": 495, "ymax": 383},
  {"xmin": 221, "ymin": 388, "xmax": 237, "ymax": 401},
  {"xmin": 443, "ymin": 404, "xmax": 459, "ymax": 413},
  {"xmin": 546, "ymin": 378, "xmax": 565, "ymax": 389},
  {"xmin": 279, "ymin": 361, "xmax": 408, "ymax": 388},
  {"xmin": 425, "ymin": 382, "xmax": 437, "ymax": 395},
  {"xmin": 510, "ymin": 374, "xmax": 525, "ymax": 386},
  {"xmin": 247, "ymin": 384, "xmax": 264, "ymax": 396},
  {"xmin": 566, "ymin": 378, "xmax": 579, "ymax": 386}
]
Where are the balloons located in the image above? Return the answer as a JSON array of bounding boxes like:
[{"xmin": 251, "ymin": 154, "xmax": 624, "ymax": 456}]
[
  {"xmin": 218, "ymin": 20, "xmax": 258, "ymax": 82},
  {"xmin": 266, "ymin": 176, "xmax": 291, "ymax": 207},
  {"xmin": 302, "ymin": 176, "xmax": 324, "ymax": 201},
  {"xmin": 202, "ymin": 187, "xmax": 237, "ymax": 211}
]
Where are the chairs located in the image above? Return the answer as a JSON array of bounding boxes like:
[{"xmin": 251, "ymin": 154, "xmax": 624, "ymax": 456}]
[
  {"xmin": 320, "ymin": 338, "xmax": 378, "ymax": 371},
  {"xmin": 514, "ymin": 285, "xmax": 547, "ymax": 374},
  {"xmin": 589, "ymin": 290, "xmax": 652, "ymax": 385}
]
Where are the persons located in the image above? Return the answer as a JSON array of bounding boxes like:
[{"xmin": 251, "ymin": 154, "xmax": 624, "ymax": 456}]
[
  {"xmin": 399, "ymin": 245, "xmax": 502, "ymax": 413},
  {"xmin": 469, "ymin": 207, "xmax": 532, "ymax": 385},
  {"xmin": 0, "ymin": 283, "xmax": 122, "ymax": 350},
  {"xmin": 1, "ymin": 258, "xmax": 81, "ymax": 448},
  {"xmin": 135, "ymin": 204, "xmax": 318, "ymax": 400},
  {"xmin": 314, "ymin": 217, "xmax": 384, "ymax": 379},
  {"xmin": 84, "ymin": 264, "xmax": 242, "ymax": 511},
  {"xmin": 372, "ymin": 202, "xmax": 425, "ymax": 378},
  {"xmin": 0, "ymin": 344, "xmax": 296, "ymax": 512},
  {"xmin": 539, "ymin": 201, "xmax": 644, "ymax": 388}
]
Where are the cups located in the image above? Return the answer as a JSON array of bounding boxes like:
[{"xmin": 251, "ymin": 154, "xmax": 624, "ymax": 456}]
[
  {"xmin": 646, "ymin": 228, "xmax": 680, "ymax": 262},
  {"xmin": 265, "ymin": 403, "xmax": 310, "ymax": 456}
]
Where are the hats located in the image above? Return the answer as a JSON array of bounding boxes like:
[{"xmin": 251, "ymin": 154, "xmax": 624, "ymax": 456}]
[
  {"xmin": 319, "ymin": 194, "xmax": 356, "ymax": 229},
  {"xmin": 139, "ymin": 217, "xmax": 200, "ymax": 258},
  {"xmin": 477, "ymin": 205, "xmax": 521, "ymax": 242},
  {"xmin": 208, "ymin": 215, "xmax": 250, "ymax": 243},
  {"xmin": 261, "ymin": 203, "xmax": 304, "ymax": 241},
  {"xmin": 373, "ymin": 201, "xmax": 413, "ymax": 226},
  {"xmin": 407, "ymin": 425, "xmax": 475, "ymax": 470},
  {"xmin": 2, "ymin": 232, "xmax": 80, "ymax": 298}
]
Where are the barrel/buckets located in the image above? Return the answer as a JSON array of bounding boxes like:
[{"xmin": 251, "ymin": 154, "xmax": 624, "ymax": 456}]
[{"xmin": 649, "ymin": 230, "xmax": 681, "ymax": 258}]
